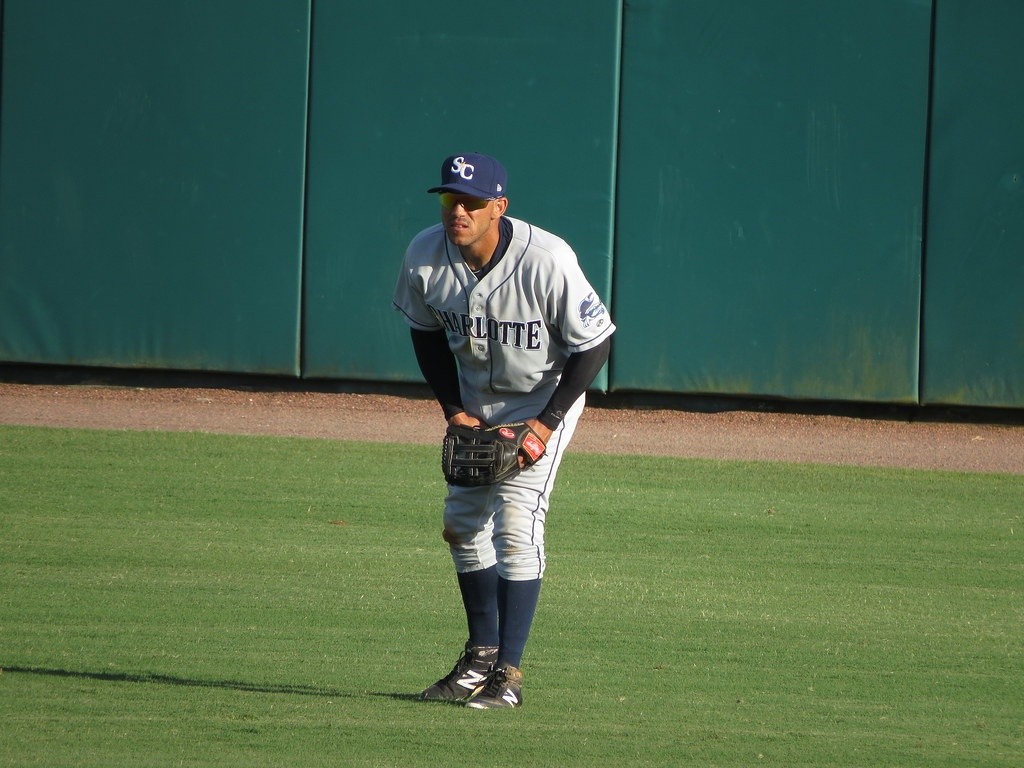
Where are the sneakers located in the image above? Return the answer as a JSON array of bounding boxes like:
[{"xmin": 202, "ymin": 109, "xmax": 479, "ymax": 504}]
[{"xmin": 420, "ymin": 641, "xmax": 523, "ymax": 710}]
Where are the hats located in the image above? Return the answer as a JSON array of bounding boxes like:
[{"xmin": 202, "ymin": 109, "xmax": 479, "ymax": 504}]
[{"xmin": 427, "ymin": 152, "xmax": 507, "ymax": 198}]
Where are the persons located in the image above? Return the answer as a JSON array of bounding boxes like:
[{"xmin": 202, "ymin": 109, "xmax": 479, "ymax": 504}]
[{"xmin": 392, "ymin": 151, "xmax": 616, "ymax": 712}]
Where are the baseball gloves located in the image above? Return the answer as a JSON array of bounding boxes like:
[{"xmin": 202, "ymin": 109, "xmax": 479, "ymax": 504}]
[{"xmin": 440, "ymin": 421, "xmax": 549, "ymax": 488}]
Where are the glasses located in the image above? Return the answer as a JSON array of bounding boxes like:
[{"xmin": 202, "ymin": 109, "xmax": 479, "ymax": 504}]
[{"xmin": 439, "ymin": 192, "xmax": 489, "ymax": 210}]
